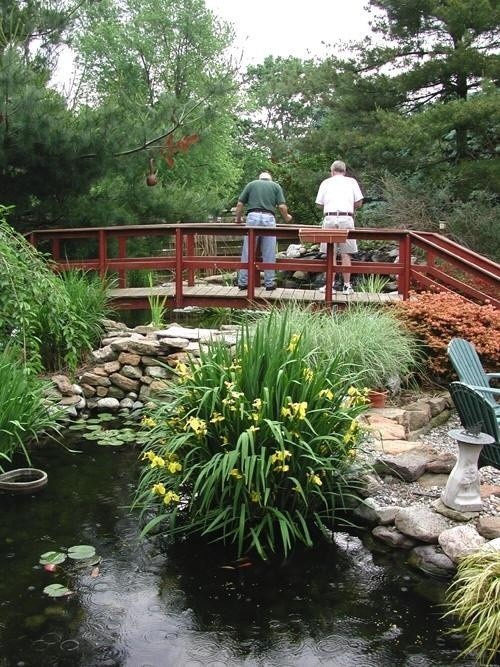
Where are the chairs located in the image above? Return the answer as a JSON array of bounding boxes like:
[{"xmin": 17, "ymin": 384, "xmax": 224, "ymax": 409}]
[
  {"xmin": 448, "ymin": 381, "xmax": 500, "ymax": 471},
  {"xmin": 447, "ymin": 338, "xmax": 500, "ymax": 417}
]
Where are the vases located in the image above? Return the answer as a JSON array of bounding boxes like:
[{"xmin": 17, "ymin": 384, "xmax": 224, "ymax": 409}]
[{"xmin": 368, "ymin": 388, "xmax": 389, "ymax": 408}]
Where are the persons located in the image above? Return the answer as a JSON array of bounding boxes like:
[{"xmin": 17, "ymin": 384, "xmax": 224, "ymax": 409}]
[
  {"xmin": 234, "ymin": 171, "xmax": 292, "ymax": 290},
  {"xmin": 314, "ymin": 158, "xmax": 365, "ymax": 295}
]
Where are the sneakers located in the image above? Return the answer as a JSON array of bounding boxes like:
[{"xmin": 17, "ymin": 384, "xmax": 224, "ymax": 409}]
[
  {"xmin": 343, "ymin": 284, "xmax": 354, "ymax": 295},
  {"xmin": 319, "ymin": 285, "xmax": 337, "ymax": 295},
  {"xmin": 239, "ymin": 285, "xmax": 247, "ymax": 289},
  {"xmin": 266, "ymin": 287, "xmax": 275, "ymax": 290}
]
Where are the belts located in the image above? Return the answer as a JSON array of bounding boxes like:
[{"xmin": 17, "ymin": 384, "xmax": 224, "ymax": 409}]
[{"xmin": 324, "ymin": 212, "xmax": 354, "ymax": 216}]
